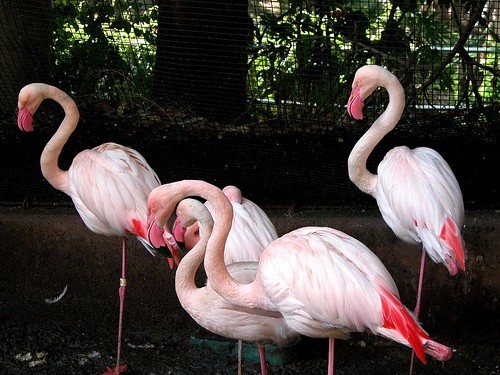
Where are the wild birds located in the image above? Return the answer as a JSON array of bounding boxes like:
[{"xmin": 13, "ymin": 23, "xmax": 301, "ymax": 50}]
[
  {"xmin": 17, "ymin": 82, "xmax": 179, "ymax": 375},
  {"xmin": 346, "ymin": 64, "xmax": 469, "ymax": 375},
  {"xmin": 172, "ymin": 198, "xmax": 300, "ymax": 375},
  {"xmin": 145, "ymin": 179, "xmax": 454, "ymax": 375},
  {"xmin": 184, "ymin": 185, "xmax": 279, "ymax": 375}
]
